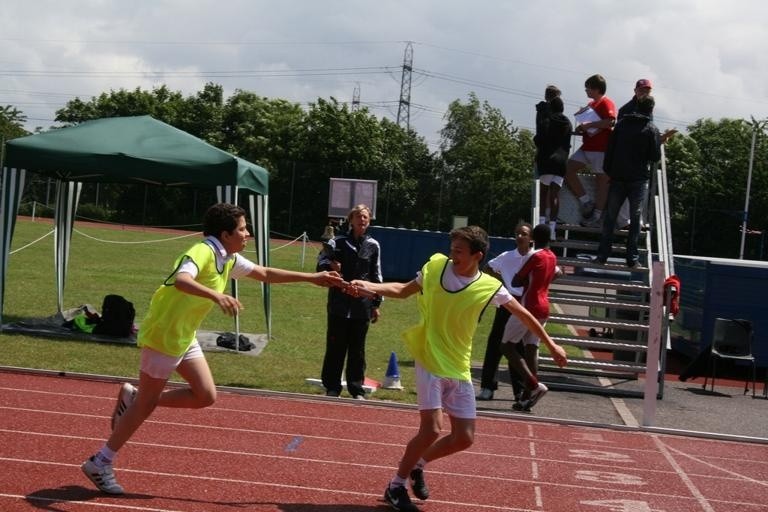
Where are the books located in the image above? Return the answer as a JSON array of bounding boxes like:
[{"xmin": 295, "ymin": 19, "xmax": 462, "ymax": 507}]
[{"xmin": 573, "ymin": 106, "xmax": 606, "ymax": 138}]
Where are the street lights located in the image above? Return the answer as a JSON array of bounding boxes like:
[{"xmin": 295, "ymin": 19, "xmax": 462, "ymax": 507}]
[{"xmin": 739, "ymin": 114, "xmax": 768, "ymax": 260}]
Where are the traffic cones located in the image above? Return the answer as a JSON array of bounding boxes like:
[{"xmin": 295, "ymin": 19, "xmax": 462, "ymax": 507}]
[{"xmin": 381, "ymin": 352, "xmax": 405, "ymax": 390}]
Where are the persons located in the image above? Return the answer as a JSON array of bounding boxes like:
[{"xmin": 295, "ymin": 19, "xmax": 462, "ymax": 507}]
[
  {"xmin": 567, "ymin": 75, "xmax": 616, "ymax": 229},
  {"xmin": 476, "ymin": 224, "xmax": 563, "ymax": 404},
  {"xmin": 347, "ymin": 224, "xmax": 568, "ymax": 510},
  {"xmin": 529, "ymin": 86, "xmax": 566, "ymax": 223},
  {"xmin": 316, "ymin": 205, "xmax": 385, "ymax": 401},
  {"xmin": 615, "ymin": 78, "xmax": 678, "ymax": 229},
  {"xmin": 78, "ymin": 201, "xmax": 344, "ymax": 495},
  {"xmin": 532, "ymin": 97, "xmax": 574, "ymax": 242},
  {"xmin": 594, "ymin": 95, "xmax": 662, "ymax": 267},
  {"xmin": 499, "ymin": 224, "xmax": 559, "ymax": 411}
]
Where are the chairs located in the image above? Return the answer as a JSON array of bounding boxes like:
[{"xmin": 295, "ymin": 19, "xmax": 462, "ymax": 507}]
[{"xmin": 696, "ymin": 316, "xmax": 758, "ymax": 399}]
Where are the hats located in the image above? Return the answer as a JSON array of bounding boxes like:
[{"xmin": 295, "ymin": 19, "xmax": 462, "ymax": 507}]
[{"xmin": 636, "ymin": 80, "xmax": 652, "ymax": 89}]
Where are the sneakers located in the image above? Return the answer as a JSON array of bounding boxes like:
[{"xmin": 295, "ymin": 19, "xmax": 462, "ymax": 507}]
[
  {"xmin": 627, "ymin": 260, "xmax": 642, "ymax": 267},
  {"xmin": 111, "ymin": 383, "xmax": 139, "ymax": 431},
  {"xmin": 580, "ymin": 215, "xmax": 600, "ymax": 228},
  {"xmin": 384, "ymin": 486, "xmax": 420, "ymax": 512},
  {"xmin": 409, "ymin": 468, "xmax": 429, "ymax": 500},
  {"xmin": 545, "ymin": 216, "xmax": 564, "ymax": 224},
  {"xmin": 476, "ymin": 382, "xmax": 548, "ymax": 411},
  {"xmin": 591, "ymin": 256, "xmax": 608, "ymax": 265},
  {"xmin": 80, "ymin": 455, "xmax": 124, "ymax": 493},
  {"xmin": 581, "ymin": 200, "xmax": 595, "ymax": 218}
]
